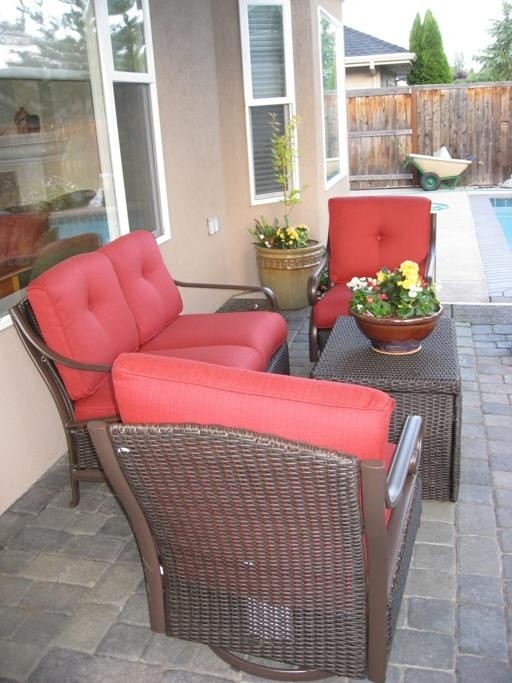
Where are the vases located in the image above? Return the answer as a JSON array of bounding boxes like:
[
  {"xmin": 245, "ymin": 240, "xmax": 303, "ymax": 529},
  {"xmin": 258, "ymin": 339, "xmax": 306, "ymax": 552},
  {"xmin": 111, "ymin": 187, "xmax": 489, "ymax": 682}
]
[
  {"xmin": 349, "ymin": 312, "xmax": 442, "ymax": 356},
  {"xmin": 255, "ymin": 240, "xmax": 325, "ymax": 312}
]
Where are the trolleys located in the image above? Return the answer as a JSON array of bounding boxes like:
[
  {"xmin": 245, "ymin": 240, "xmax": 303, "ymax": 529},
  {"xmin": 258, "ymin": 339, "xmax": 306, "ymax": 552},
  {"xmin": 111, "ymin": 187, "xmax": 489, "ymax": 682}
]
[{"xmin": 392, "ymin": 132, "xmax": 472, "ymax": 191}]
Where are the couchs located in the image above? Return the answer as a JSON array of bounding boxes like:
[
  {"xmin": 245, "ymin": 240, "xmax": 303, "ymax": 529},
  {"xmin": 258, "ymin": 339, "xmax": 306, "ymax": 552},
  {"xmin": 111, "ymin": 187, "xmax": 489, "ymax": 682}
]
[{"xmin": 10, "ymin": 230, "xmax": 291, "ymax": 508}]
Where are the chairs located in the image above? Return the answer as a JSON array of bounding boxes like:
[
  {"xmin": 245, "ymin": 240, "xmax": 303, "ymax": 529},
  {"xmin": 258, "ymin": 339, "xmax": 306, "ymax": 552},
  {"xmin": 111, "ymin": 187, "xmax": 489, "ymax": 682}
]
[
  {"xmin": 87, "ymin": 355, "xmax": 423, "ymax": 683},
  {"xmin": 0, "ymin": 211, "xmax": 100, "ymax": 296},
  {"xmin": 306, "ymin": 195, "xmax": 437, "ymax": 358}
]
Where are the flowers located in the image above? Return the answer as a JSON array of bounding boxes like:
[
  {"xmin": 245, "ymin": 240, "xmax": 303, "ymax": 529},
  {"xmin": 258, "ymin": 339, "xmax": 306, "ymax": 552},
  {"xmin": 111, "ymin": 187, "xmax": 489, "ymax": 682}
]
[
  {"xmin": 249, "ymin": 114, "xmax": 308, "ymax": 249},
  {"xmin": 346, "ymin": 260, "xmax": 437, "ymax": 317}
]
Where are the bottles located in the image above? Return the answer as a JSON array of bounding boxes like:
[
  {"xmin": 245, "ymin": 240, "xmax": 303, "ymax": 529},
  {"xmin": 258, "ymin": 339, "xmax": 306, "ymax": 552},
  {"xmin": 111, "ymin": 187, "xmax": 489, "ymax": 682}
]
[{"xmin": 15, "ymin": 106, "xmax": 31, "ymax": 133}]
